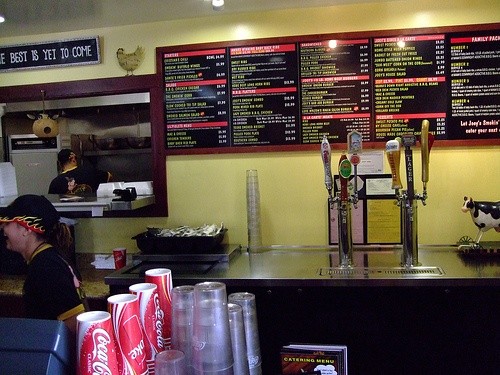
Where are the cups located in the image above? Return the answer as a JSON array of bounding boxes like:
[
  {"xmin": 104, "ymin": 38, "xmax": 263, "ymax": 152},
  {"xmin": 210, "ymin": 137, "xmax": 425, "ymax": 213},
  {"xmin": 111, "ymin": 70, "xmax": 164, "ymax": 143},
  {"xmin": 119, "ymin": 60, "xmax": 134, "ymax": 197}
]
[
  {"xmin": 76, "ymin": 269, "xmax": 262, "ymax": 375},
  {"xmin": 245, "ymin": 169, "xmax": 262, "ymax": 253},
  {"xmin": 113, "ymin": 248, "xmax": 127, "ymax": 269}
]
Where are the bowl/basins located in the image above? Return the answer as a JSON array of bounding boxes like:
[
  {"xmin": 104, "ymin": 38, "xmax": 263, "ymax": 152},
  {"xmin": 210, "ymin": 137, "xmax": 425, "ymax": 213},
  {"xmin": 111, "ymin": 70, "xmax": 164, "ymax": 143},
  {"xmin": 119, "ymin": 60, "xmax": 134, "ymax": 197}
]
[{"xmin": 96, "ymin": 181, "xmax": 153, "ymax": 198}]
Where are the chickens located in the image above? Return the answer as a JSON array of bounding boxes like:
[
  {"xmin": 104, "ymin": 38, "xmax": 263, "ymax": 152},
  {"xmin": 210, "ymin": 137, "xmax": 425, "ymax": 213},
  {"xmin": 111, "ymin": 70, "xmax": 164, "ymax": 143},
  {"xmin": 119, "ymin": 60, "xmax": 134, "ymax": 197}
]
[{"xmin": 117, "ymin": 45, "xmax": 145, "ymax": 77}]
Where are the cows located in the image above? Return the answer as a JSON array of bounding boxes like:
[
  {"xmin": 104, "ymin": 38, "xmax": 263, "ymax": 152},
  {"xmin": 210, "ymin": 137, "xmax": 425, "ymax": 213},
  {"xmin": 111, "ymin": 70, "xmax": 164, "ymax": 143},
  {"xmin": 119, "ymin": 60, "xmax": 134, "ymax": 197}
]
[{"xmin": 462, "ymin": 196, "xmax": 500, "ymax": 245}]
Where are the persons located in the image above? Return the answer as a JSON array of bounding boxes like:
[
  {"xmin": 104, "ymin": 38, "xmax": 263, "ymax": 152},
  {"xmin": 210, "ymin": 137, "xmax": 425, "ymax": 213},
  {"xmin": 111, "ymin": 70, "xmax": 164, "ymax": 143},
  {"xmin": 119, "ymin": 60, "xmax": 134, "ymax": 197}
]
[
  {"xmin": 0, "ymin": 194, "xmax": 80, "ymax": 336},
  {"xmin": 48, "ymin": 149, "xmax": 112, "ymax": 197}
]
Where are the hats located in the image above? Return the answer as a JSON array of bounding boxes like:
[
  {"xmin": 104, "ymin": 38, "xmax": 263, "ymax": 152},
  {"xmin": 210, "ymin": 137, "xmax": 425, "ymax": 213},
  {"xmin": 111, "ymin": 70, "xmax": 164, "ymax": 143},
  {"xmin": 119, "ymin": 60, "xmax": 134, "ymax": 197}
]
[
  {"xmin": 58, "ymin": 148, "xmax": 77, "ymax": 164},
  {"xmin": 0, "ymin": 194, "xmax": 60, "ymax": 236}
]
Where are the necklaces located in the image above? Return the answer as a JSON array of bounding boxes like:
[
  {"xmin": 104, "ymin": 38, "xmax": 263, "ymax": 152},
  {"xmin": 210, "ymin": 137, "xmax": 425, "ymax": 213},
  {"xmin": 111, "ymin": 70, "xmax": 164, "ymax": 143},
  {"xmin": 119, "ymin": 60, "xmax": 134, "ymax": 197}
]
[{"xmin": 31, "ymin": 241, "xmax": 45, "ymax": 256}]
